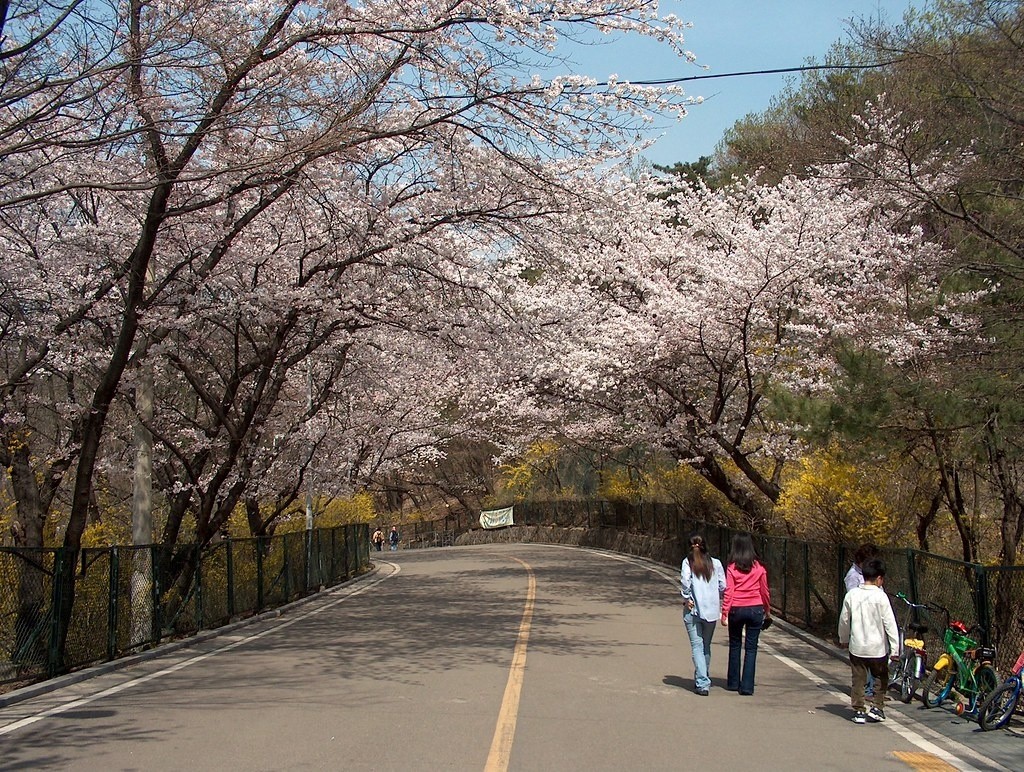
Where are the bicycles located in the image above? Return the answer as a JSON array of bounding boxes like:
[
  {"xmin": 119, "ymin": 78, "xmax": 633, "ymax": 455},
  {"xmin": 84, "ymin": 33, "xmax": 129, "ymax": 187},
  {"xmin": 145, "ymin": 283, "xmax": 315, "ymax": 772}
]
[
  {"xmin": 977, "ymin": 649, "xmax": 1024, "ymax": 737},
  {"xmin": 922, "ymin": 600, "xmax": 1001, "ymax": 724},
  {"xmin": 885, "ymin": 591, "xmax": 938, "ymax": 704}
]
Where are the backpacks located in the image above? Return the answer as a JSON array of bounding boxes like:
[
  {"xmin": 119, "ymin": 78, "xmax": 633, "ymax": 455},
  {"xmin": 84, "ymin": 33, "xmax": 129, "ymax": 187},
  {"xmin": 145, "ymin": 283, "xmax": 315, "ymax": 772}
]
[
  {"xmin": 392, "ymin": 532, "xmax": 398, "ymax": 541},
  {"xmin": 375, "ymin": 532, "xmax": 381, "ymax": 543}
]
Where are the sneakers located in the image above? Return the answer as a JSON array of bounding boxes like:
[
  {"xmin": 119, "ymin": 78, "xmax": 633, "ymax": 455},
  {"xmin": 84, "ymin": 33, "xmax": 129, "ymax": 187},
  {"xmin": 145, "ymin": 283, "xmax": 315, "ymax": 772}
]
[
  {"xmin": 850, "ymin": 712, "xmax": 866, "ymax": 724},
  {"xmin": 868, "ymin": 708, "xmax": 887, "ymax": 722}
]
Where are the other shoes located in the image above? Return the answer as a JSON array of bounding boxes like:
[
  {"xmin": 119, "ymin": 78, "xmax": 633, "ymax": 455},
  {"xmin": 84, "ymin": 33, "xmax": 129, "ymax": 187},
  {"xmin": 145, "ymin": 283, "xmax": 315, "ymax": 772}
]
[{"xmin": 693, "ymin": 688, "xmax": 709, "ymax": 696}]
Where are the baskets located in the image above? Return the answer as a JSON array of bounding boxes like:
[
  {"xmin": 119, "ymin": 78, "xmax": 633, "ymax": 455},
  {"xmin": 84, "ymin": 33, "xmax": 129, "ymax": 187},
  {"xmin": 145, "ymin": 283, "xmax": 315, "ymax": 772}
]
[{"xmin": 943, "ymin": 630, "xmax": 976, "ymax": 656}]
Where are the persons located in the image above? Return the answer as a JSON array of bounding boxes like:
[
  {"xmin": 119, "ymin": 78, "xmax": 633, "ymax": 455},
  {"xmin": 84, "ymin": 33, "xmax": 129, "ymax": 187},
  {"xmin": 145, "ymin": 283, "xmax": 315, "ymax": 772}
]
[
  {"xmin": 680, "ymin": 534, "xmax": 727, "ymax": 695},
  {"xmin": 837, "ymin": 543, "xmax": 899, "ymax": 724},
  {"xmin": 722, "ymin": 531, "xmax": 773, "ymax": 695},
  {"xmin": 372, "ymin": 527, "xmax": 385, "ymax": 551},
  {"xmin": 389, "ymin": 525, "xmax": 400, "ymax": 551}
]
